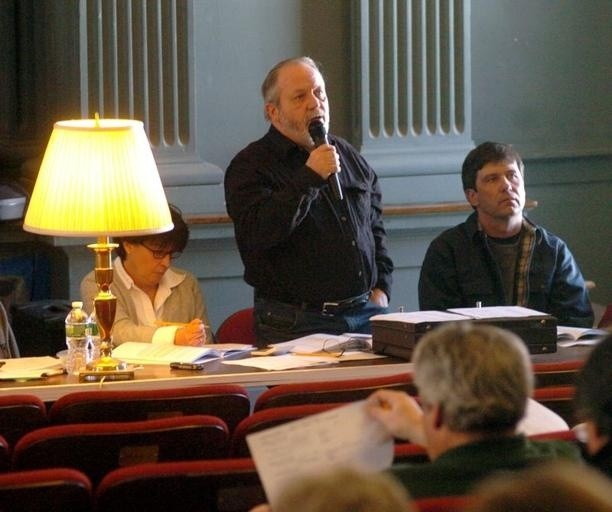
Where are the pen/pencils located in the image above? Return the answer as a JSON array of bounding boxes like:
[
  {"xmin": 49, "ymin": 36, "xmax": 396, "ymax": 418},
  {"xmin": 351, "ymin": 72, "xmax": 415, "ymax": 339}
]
[
  {"xmin": 171, "ymin": 361, "xmax": 202, "ymax": 371},
  {"xmin": 40, "ymin": 369, "xmax": 69, "ymax": 377},
  {"xmin": 154, "ymin": 319, "xmax": 211, "ymax": 330}
]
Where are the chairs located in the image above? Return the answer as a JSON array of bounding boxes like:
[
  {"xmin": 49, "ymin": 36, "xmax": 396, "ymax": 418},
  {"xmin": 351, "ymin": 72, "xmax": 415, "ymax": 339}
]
[
  {"xmin": 50, "ymin": 384, "xmax": 252, "ymax": 431},
  {"xmin": 215, "ymin": 306, "xmax": 255, "ymax": 347},
  {"xmin": 533, "ymin": 387, "xmax": 577, "ymax": 423},
  {"xmin": 100, "ymin": 456, "xmax": 268, "ymax": 511},
  {"xmin": 1, "ymin": 300, "xmax": 20, "ymax": 356},
  {"xmin": 406, "ymin": 495, "xmax": 486, "ymax": 511},
  {"xmin": 530, "ymin": 358, "xmax": 586, "ymax": 387},
  {"xmin": 230, "ymin": 409, "xmax": 325, "ymax": 459},
  {"xmin": 255, "ymin": 372, "xmax": 420, "ymax": 411},
  {"xmin": 3, "ymin": 468, "xmax": 94, "ymax": 510},
  {"xmin": 389, "ymin": 429, "xmax": 581, "ymax": 457},
  {"xmin": 13, "ymin": 414, "xmax": 233, "ymax": 486},
  {"xmin": 1, "ymin": 394, "xmax": 47, "ymax": 449}
]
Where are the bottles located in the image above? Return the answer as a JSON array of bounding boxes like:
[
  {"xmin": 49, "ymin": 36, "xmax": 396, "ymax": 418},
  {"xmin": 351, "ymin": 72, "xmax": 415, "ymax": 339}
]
[
  {"xmin": 87, "ymin": 299, "xmax": 104, "ymax": 370},
  {"xmin": 64, "ymin": 300, "xmax": 89, "ymax": 376}
]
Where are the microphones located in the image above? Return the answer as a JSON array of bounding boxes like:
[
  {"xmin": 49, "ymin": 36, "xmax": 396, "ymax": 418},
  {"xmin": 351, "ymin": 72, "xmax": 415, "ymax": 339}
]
[{"xmin": 308, "ymin": 120, "xmax": 344, "ymax": 201}]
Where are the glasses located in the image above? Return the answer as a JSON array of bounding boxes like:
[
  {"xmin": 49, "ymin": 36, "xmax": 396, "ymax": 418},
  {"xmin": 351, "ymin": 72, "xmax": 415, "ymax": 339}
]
[
  {"xmin": 140, "ymin": 243, "xmax": 184, "ymax": 259},
  {"xmin": 323, "ymin": 337, "xmax": 372, "ymax": 357}
]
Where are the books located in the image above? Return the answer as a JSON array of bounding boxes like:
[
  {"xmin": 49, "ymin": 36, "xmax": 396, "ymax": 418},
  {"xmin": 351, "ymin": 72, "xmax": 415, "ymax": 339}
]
[
  {"xmin": 556, "ymin": 325, "xmax": 611, "ymax": 341},
  {"xmin": 111, "ymin": 338, "xmax": 258, "ymax": 364},
  {"xmin": 0, "ymin": 355, "xmax": 64, "ymax": 380},
  {"xmin": 269, "ymin": 332, "xmax": 372, "ymax": 351}
]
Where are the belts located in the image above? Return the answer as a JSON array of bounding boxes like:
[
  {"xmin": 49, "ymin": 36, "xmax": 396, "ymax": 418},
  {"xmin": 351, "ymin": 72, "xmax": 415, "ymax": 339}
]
[{"xmin": 255, "ymin": 288, "xmax": 370, "ymax": 313}]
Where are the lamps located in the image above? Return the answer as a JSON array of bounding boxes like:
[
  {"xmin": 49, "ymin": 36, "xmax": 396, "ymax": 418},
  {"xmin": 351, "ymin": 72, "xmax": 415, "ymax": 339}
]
[{"xmin": 21, "ymin": 116, "xmax": 175, "ymax": 381}]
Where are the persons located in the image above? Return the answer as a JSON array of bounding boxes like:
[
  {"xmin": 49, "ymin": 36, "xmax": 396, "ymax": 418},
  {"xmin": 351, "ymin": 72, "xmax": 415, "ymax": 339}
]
[
  {"xmin": 80, "ymin": 203, "xmax": 211, "ymax": 348},
  {"xmin": 569, "ymin": 331, "xmax": 611, "ymax": 483},
  {"xmin": 358, "ymin": 317, "xmax": 566, "ymax": 494},
  {"xmin": 471, "ymin": 465, "xmax": 611, "ymax": 512},
  {"xmin": 251, "ymin": 467, "xmax": 422, "ymax": 512},
  {"xmin": 416, "ymin": 140, "xmax": 595, "ymax": 329},
  {"xmin": 223, "ymin": 53, "xmax": 394, "ymax": 342}
]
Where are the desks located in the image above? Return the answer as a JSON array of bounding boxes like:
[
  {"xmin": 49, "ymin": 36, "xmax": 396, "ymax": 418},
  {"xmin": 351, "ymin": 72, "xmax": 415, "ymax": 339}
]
[{"xmin": 0, "ymin": 323, "xmax": 612, "ymax": 405}]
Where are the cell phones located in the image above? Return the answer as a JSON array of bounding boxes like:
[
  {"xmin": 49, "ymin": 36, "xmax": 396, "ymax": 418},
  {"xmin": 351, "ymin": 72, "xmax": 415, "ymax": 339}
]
[{"xmin": 170, "ymin": 362, "xmax": 203, "ymax": 370}]
